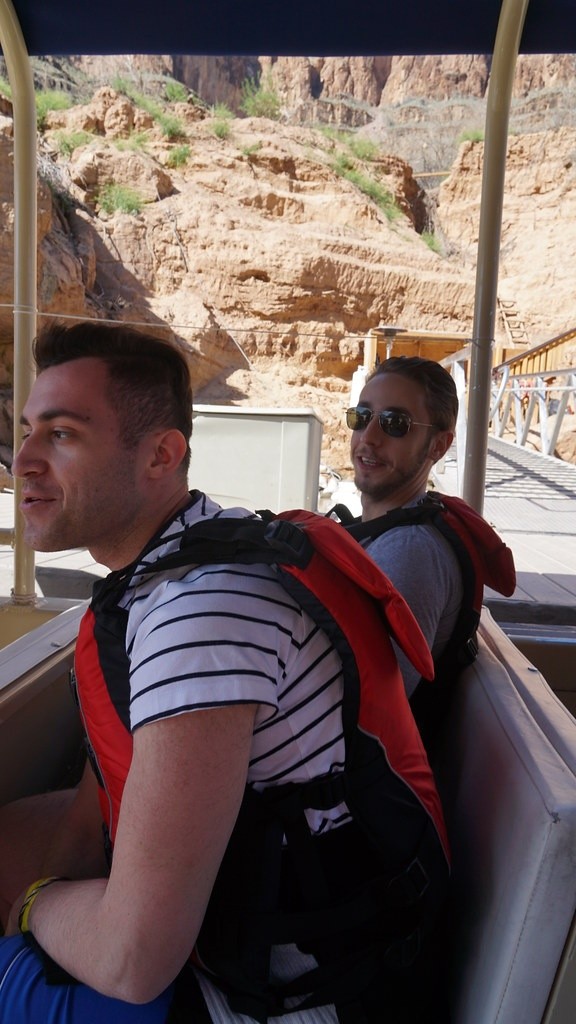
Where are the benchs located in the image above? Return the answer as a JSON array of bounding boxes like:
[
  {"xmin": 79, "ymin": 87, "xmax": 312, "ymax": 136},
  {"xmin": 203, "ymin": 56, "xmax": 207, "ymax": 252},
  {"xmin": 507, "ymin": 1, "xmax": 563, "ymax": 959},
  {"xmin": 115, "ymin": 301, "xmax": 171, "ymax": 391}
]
[
  {"xmin": 322, "ymin": 601, "xmax": 576, "ymax": 1024},
  {"xmin": 0, "ymin": 596, "xmax": 97, "ymax": 803}
]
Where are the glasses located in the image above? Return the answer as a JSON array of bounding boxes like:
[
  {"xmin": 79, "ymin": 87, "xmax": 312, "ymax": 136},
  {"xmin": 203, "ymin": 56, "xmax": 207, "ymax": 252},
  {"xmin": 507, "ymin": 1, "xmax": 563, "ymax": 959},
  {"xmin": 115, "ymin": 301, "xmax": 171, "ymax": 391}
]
[{"xmin": 345, "ymin": 406, "xmax": 434, "ymax": 438}]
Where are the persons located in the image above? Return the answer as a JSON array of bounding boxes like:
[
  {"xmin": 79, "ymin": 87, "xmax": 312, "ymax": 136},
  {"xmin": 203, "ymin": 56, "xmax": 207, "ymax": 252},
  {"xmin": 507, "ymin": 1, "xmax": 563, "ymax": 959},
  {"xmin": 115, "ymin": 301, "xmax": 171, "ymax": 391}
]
[
  {"xmin": 0, "ymin": 321, "xmax": 464, "ymax": 1024},
  {"xmin": 325, "ymin": 355, "xmax": 515, "ymax": 829}
]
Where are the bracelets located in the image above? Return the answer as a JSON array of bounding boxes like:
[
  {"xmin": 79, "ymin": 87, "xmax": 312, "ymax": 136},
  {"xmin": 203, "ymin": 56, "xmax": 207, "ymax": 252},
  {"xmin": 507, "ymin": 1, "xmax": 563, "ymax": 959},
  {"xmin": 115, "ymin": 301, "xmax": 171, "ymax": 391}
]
[{"xmin": 18, "ymin": 876, "xmax": 71, "ymax": 932}]
